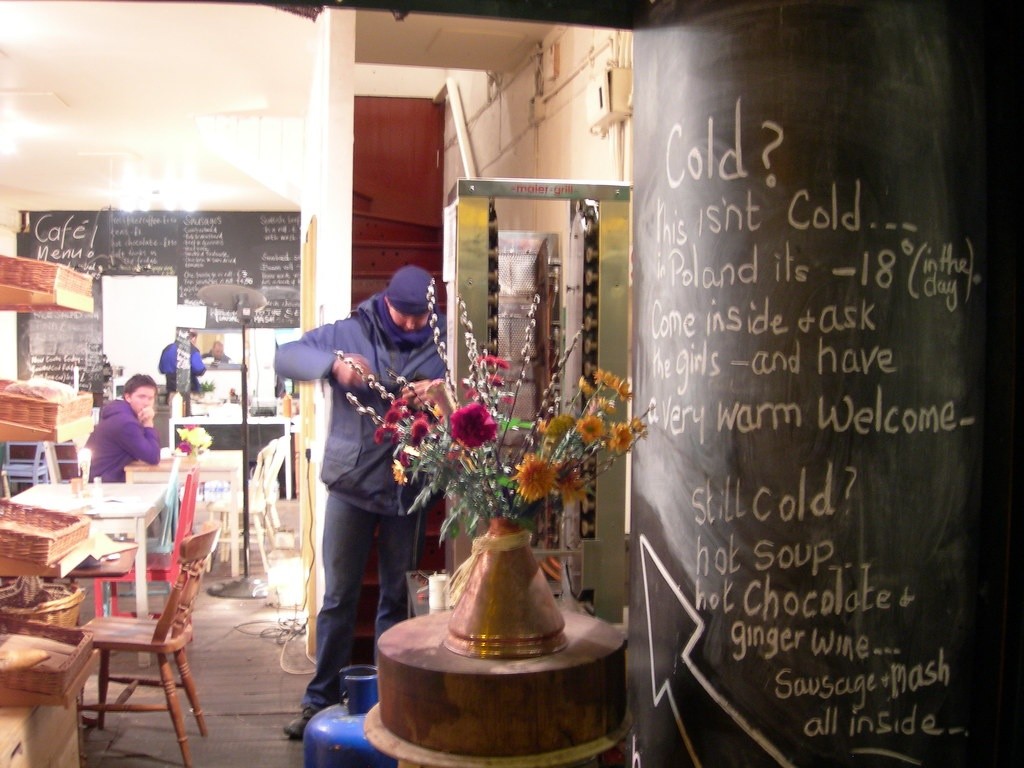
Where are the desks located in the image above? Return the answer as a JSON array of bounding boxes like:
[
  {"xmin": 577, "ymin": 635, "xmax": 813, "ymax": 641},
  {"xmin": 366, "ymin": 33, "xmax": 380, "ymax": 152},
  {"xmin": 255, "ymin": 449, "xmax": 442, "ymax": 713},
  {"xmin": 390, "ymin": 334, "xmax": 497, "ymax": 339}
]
[
  {"xmin": 8, "ymin": 481, "xmax": 178, "ymax": 668},
  {"xmin": 124, "ymin": 448, "xmax": 243, "ymax": 577}
]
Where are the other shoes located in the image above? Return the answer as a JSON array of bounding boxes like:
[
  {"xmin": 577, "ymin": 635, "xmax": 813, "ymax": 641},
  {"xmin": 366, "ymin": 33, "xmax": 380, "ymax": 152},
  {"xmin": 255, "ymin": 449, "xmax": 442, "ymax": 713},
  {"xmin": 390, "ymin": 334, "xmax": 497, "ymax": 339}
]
[{"xmin": 283, "ymin": 703, "xmax": 321, "ymax": 737}]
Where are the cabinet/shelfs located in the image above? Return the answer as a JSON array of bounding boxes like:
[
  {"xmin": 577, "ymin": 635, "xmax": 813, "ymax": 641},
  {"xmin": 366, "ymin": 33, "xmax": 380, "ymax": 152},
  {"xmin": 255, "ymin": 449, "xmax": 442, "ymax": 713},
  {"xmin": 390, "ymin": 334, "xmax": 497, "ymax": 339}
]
[{"xmin": 0, "ymin": 284, "xmax": 95, "ymax": 768}]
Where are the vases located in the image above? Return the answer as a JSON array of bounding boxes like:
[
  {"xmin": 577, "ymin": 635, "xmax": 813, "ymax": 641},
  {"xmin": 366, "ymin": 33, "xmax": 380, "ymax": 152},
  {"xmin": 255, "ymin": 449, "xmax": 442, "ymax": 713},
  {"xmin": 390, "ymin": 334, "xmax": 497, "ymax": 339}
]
[
  {"xmin": 188, "ymin": 454, "xmax": 197, "ymax": 467},
  {"xmin": 444, "ymin": 513, "xmax": 571, "ymax": 661}
]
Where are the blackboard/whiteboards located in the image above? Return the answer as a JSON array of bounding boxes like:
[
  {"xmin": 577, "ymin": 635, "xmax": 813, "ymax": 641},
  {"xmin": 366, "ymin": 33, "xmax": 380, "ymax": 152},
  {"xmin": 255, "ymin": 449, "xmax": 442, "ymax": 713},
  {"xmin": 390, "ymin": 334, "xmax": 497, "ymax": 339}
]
[
  {"xmin": 18, "ymin": 209, "xmax": 300, "ymax": 393},
  {"xmin": 630, "ymin": 0, "xmax": 1023, "ymax": 768}
]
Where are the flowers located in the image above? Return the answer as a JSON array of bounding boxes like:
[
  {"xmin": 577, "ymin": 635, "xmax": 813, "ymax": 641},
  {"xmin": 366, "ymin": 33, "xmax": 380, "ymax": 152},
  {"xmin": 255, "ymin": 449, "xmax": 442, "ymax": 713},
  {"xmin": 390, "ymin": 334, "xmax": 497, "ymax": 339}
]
[
  {"xmin": 332, "ymin": 277, "xmax": 658, "ymax": 544},
  {"xmin": 173, "ymin": 423, "xmax": 213, "ymax": 457}
]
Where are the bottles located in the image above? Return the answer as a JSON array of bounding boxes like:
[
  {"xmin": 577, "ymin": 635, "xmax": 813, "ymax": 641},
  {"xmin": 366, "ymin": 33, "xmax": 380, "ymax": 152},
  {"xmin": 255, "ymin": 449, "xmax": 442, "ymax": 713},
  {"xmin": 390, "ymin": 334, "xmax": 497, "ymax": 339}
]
[{"xmin": 91, "ymin": 477, "xmax": 103, "ymax": 508}]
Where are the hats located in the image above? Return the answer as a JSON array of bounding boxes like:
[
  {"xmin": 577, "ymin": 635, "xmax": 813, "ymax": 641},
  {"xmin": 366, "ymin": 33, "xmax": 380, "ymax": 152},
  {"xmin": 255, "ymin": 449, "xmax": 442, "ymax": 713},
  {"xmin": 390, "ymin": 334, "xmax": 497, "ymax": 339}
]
[{"xmin": 386, "ymin": 265, "xmax": 437, "ymax": 315}]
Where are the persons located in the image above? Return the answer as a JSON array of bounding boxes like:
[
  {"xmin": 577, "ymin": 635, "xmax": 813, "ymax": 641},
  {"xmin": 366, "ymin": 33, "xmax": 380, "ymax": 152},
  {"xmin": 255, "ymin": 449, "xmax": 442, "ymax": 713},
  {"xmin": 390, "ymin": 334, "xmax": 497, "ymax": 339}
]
[
  {"xmin": 84, "ymin": 374, "xmax": 161, "ymax": 483},
  {"xmin": 157, "ymin": 333, "xmax": 207, "ymax": 403},
  {"xmin": 273, "ymin": 264, "xmax": 448, "ymax": 737},
  {"xmin": 201, "ymin": 341, "xmax": 231, "ymax": 364}
]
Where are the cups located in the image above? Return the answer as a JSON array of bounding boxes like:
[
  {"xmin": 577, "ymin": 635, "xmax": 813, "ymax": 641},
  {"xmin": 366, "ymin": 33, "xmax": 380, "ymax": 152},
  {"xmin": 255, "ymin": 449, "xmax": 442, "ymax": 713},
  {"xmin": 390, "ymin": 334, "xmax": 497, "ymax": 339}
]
[{"xmin": 72, "ymin": 477, "xmax": 83, "ymax": 493}]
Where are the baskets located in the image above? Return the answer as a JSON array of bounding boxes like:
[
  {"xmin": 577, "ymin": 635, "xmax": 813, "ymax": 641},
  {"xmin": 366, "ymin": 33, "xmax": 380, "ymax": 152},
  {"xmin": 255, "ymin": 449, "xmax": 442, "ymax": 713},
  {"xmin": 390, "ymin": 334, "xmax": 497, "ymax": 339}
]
[
  {"xmin": 0, "ymin": 379, "xmax": 94, "ymax": 430},
  {"xmin": 0, "ymin": 616, "xmax": 94, "ymax": 693},
  {"xmin": 0, "ymin": 580, "xmax": 85, "ymax": 628},
  {"xmin": 0, "ymin": 499, "xmax": 90, "ymax": 568},
  {"xmin": 1, "ymin": 254, "xmax": 93, "ymax": 298}
]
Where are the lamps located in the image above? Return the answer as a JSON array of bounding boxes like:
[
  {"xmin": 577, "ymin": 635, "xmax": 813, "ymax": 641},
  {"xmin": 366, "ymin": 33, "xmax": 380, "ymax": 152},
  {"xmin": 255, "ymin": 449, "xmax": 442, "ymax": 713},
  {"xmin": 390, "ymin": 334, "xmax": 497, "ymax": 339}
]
[
  {"xmin": 199, "ymin": 284, "xmax": 269, "ymax": 599},
  {"xmin": 265, "ymin": 556, "xmax": 304, "ymax": 606}
]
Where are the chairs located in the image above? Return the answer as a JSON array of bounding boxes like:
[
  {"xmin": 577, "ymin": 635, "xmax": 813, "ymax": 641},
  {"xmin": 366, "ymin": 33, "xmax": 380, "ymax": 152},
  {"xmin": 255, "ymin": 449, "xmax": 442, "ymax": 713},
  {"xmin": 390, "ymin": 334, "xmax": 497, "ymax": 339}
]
[
  {"xmin": 197, "ymin": 435, "xmax": 292, "ymax": 576},
  {"xmin": 96, "ymin": 465, "xmax": 200, "ymax": 644},
  {"xmin": 6, "ymin": 442, "xmax": 50, "ymax": 486},
  {"xmin": 71, "ymin": 522, "xmax": 221, "ymax": 768}
]
